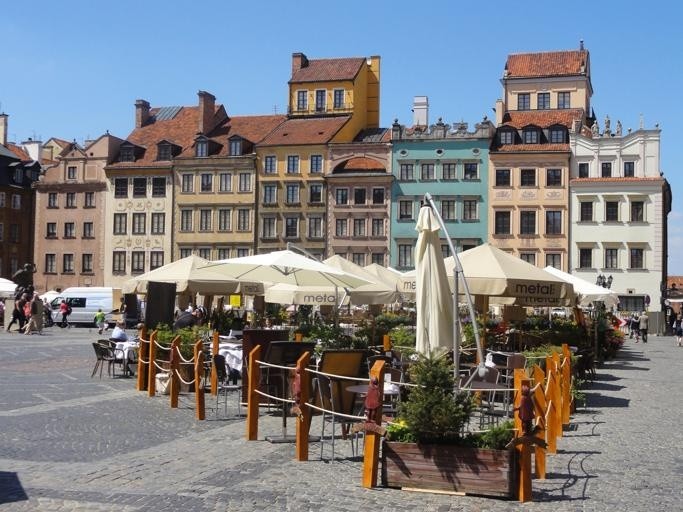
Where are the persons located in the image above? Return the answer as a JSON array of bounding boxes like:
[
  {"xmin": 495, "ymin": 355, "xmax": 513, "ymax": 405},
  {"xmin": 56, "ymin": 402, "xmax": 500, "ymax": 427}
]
[
  {"xmin": 120, "ymin": 297, "xmax": 127, "ymax": 328},
  {"xmin": 11, "ymin": 263, "xmax": 37, "ymax": 288},
  {"xmin": 172, "ymin": 306, "xmax": 209, "ymax": 331},
  {"xmin": 96, "ymin": 308, "xmax": 105, "ymax": 334},
  {"xmin": 111, "ymin": 319, "xmax": 129, "ymax": 341},
  {"xmin": 672, "ymin": 315, "xmax": 683, "ymax": 346},
  {"xmin": 6, "ymin": 292, "xmax": 68, "ymax": 336},
  {"xmin": 628, "ymin": 311, "xmax": 649, "ymax": 344},
  {"xmin": 261, "ymin": 317, "xmax": 281, "ymax": 331}
]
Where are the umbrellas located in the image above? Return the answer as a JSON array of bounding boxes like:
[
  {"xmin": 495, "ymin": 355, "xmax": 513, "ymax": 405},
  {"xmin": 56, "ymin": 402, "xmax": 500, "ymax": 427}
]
[
  {"xmin": 543, "ymin": 265, "xmax": 620, "ymax": 357},
  {"xmin": 264, "ymin": 255, "xmax": 403, "ymax": 307},
  {"xmin": 414, "ymin": 192, "xmax": 490, "ymax": 396},
  {"xmin": 122, "ymin": 253, "xmax": 265, "ymax": 296},
  {"xmin": 195, "ymin": 242, "xmax": 376, "ymax": 330},
  {"xmin": 461, "ymin": 295, "xmax": 576, "ymax": 351},
  {"xmin": 396, "ymin": 242, "xmax": 574, "ymax": 349},
  {"xmin": 364, "ymin": 262, "xmax": 401, "ymax": 286}
]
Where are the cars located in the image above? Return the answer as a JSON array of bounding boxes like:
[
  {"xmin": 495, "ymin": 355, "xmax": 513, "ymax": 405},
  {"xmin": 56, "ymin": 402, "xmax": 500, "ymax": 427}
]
[
  {"xmin": 226, "ymin": 308, "xmax": 255, "ymax": 329},
  {"xmin": 550, "ymin": 306, "xmax": 566, "ymax": 319}
]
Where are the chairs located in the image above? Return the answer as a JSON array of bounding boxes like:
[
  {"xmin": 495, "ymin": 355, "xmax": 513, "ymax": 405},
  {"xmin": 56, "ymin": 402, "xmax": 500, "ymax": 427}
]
[
  {"xmin": 89, "ymin": 334, "xmax": 317, "ymax": 444},
  {"xmin": 308, "ymin": 335, "xmax": 595, "ymax": 467}
]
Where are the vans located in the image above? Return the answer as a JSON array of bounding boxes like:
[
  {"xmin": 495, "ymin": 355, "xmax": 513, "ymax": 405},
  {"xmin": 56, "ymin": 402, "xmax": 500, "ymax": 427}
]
[{"xmin": 41, "ymin": 284, "xmax": 123, "ymax": 327}]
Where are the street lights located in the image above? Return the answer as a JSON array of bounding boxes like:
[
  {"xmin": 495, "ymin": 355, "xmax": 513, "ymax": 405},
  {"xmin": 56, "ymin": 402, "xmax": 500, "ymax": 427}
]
[
  {"xmin": 594, "ymin": 274, "xmax": 613, "ymax": 289},
  {"xmin": 588, "ymin": 307, "xmax": 601, "ymax": 369}
]
